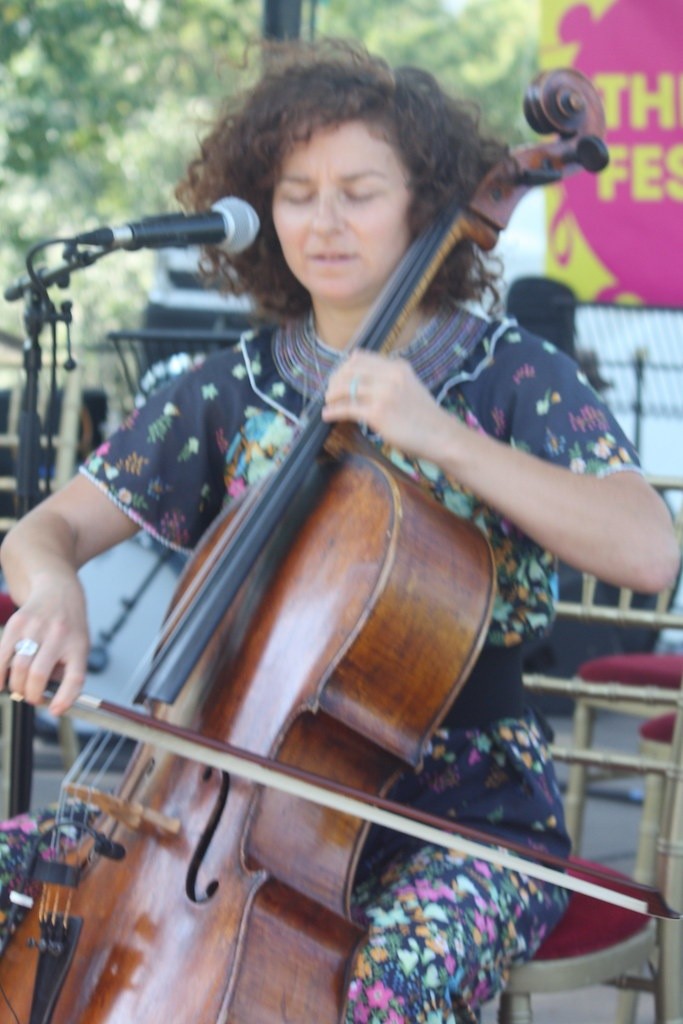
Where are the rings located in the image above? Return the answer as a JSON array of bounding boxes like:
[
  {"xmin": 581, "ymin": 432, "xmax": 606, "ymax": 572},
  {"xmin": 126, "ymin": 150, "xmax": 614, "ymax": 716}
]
[
  {"xmin": 15, "ymin": 638, "xmax": 40, "ymax": 657},
  {"xmin": 347, "ymin": 378, "xmax": 357, "ymax": 401}
]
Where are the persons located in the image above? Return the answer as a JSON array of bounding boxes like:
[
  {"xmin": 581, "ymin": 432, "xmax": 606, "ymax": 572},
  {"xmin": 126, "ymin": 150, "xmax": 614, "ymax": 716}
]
[{"xmin": 0, "ymin": 34, "xmax": 678, "ymax": 1024}]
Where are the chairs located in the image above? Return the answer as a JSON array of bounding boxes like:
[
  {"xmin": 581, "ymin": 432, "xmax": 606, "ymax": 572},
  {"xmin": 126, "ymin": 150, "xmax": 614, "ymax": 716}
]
[{"xmin": 466, "ymin": 475, "xmax": 683, "ymax": 1024}]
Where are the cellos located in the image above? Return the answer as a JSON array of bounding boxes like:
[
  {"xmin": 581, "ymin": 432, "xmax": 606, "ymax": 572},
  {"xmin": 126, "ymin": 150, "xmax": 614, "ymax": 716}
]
[{"xmin": 0, "ymin": 65, "xmax": 609, "ymax": 1024}]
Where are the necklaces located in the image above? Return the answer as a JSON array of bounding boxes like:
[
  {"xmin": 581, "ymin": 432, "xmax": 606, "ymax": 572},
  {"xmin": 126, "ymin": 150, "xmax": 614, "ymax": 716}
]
[{"xmin": 301, "ymin": 310, "xmax": 324, "ymax": 401}]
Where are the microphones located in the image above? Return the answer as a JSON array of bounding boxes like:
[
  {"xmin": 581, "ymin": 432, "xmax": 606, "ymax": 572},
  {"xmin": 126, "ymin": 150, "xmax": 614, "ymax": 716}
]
[{"xmin": 77, "ymin": 195, "xmax": 261, "ymax": 256}]
